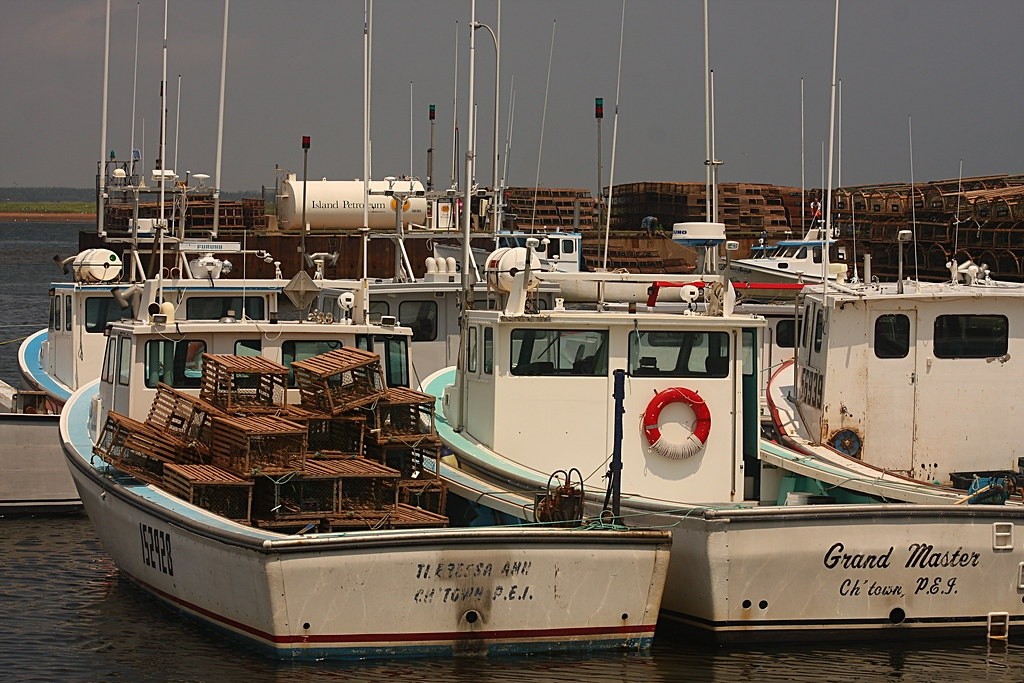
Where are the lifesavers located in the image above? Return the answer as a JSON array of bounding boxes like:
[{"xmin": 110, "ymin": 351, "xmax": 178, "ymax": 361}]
[{"xmin": 644, "ymin": 387, "xmax": 711, "ymax": 460}]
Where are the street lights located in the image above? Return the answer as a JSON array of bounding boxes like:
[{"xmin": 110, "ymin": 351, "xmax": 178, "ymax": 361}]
[{"xmin": 467, "ymin": 20, "xmax": 499, "ymax": 190}]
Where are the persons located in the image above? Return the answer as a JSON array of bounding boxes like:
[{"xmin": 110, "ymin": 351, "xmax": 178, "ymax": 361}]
[
  {"xmin": 811, "ymin": 198, "xmax": 821, "ymax": 225},
  {"xmin": 641, "ymin": 216, "xmax": 657, "ymax": 232}
]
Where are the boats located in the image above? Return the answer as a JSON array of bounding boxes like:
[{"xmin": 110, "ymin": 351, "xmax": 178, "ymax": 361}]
[{"xmin": 17, "ymin": 0, "xmax": 1024, "ymax": 663}]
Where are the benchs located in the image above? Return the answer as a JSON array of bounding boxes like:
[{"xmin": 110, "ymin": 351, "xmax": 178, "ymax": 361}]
[{"xmin": 705, "ymin": 356, "xmax": 729, "ymax": 374}]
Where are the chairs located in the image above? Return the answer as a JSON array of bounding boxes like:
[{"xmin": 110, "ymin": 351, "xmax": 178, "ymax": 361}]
[{"xmin": 530, "ymin": 362, "xmax": 554, "ymax": 376}]
